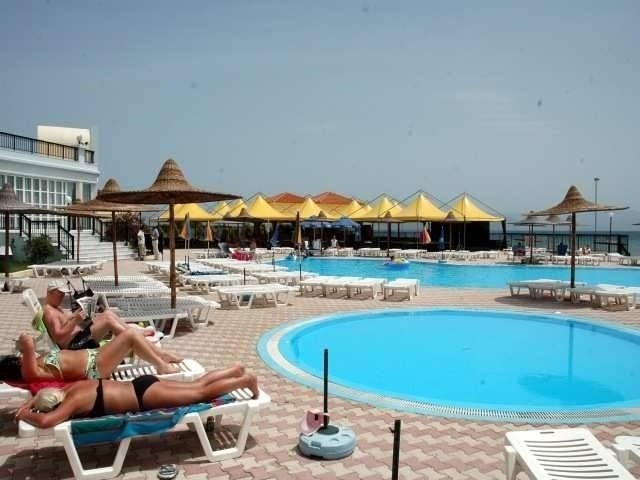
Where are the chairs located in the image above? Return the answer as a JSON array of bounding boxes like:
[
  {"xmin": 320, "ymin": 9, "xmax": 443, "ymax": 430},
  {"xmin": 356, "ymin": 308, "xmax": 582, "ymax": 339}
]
[
  {"xmin": 304, "ymin": 245, "xmax": 640, "ymax": 265},
  {"xmin": 610, "ymin": 432, "xmax": 640, "ymax": 463},
  {"xmin": 507, "ymin": 278, "xmax": 640, "ymax": 313},
  {"xmin": 17, "ymin": 385, "xmax": 273, "ymax": 480},
  {"xmin": 297, "ymin": 271, "xmax": 421, "ymax": 303},
  {"xmin": 0, "ymin": 245, "xmax": 297, "ymax": 348},
  {"xmin": 0, "ymin": 358, "xmax": 207, "ymax": 417},
  {"xmin": 501, "ymin": 426, "xmax": 638, "ymax": 480}
]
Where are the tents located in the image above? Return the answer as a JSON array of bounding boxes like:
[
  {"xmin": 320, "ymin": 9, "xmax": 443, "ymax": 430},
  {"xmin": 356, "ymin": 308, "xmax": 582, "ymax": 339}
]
[{"xmin": 160, "ymin": 190, "xmax": 505, "ymax": 251}]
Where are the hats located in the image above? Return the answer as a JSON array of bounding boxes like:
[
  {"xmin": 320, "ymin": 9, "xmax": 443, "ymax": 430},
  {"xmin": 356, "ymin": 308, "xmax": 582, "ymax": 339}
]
[
  {"xmin": 48, "ymin": 280, "xmax": 72, "ymax": 293},
  {"xmin": 298, "ymin": 408, "xmax": 329, "ymax": 436}
]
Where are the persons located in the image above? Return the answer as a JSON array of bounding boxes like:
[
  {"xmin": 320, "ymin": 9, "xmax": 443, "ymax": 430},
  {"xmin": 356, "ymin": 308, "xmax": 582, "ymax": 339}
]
[
  {"xmin": 14, "ymin": 357, "xmax": 260, "ymax": 429},
  {"xmin": 41, "ymin": 278, "xmax": 157, "ymax": 349},
  {"xmin": 0, "ymin": 331, "xmax": 187, "ymax": 382}
]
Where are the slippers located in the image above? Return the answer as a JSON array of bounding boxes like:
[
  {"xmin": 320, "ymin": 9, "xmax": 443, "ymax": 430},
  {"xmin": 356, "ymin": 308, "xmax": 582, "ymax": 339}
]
[{"xmin": 157, "ymin": 462, "xmax": 178, "ymax": 479}]
